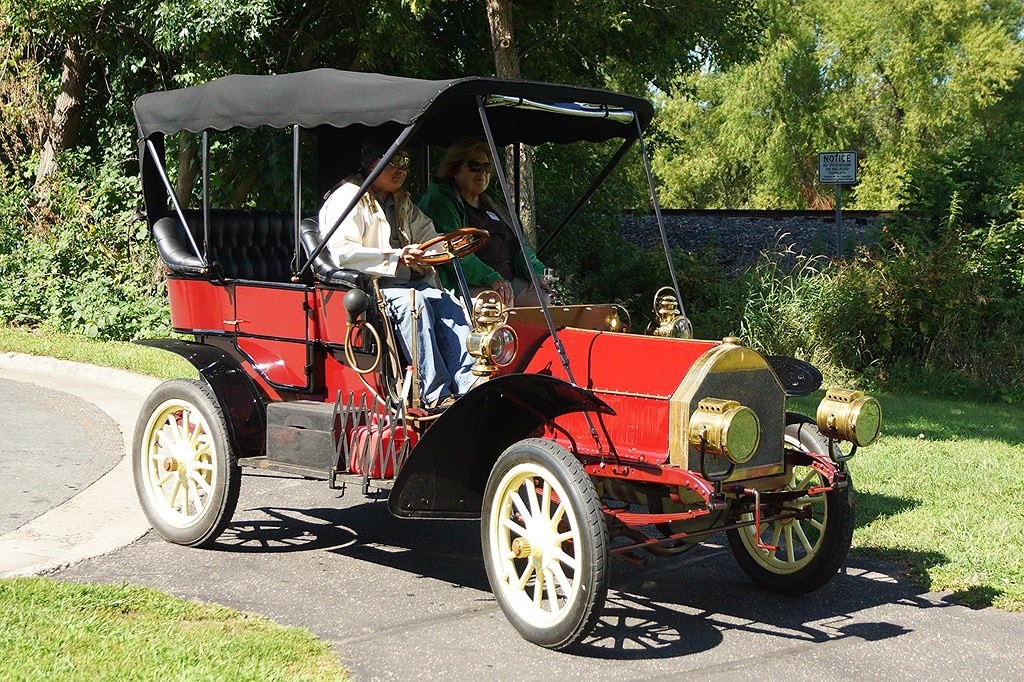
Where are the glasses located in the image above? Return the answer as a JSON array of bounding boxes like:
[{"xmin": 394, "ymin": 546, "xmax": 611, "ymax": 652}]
[
  {"xmin": 377, "ymin": 154, "xmax": 409, "ymax": 168},
  {"xmin": 467, "ymin": 160, "xmax": 494, "ymax": 173}
]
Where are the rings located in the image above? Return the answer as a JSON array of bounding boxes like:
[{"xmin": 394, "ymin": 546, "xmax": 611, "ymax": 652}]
[{"xmin": 500, "ymin": 286, "xmax": 503, "ymax": 290}]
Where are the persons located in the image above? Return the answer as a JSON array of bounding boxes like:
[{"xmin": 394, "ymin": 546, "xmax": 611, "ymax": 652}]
[
  {"xmin": 317, "ymin": 134, "xmax": 489, "ymax": 411},
  {"xmin": 418, "ymin": 138, "xmax": 551, "ymax": 308}
]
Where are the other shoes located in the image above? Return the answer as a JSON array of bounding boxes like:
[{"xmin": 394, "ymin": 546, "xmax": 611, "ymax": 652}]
[{"xmin": 425, "ymin": 395, "xmax": 456, "ymax": 412}]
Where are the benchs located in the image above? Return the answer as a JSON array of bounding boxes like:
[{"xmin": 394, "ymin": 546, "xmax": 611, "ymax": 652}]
[
  {"xmin": 299, "ymin": 215, "xmax": 388, "ymax": 356},
  {"xmin": 152, "ymin": 208, "xmax": 312, "ymax": 337}
]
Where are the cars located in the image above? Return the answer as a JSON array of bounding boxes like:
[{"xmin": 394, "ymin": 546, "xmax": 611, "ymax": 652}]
[{"xmin": 126, "ymin": 67, "xmax": 885, "ymax": 656}]
